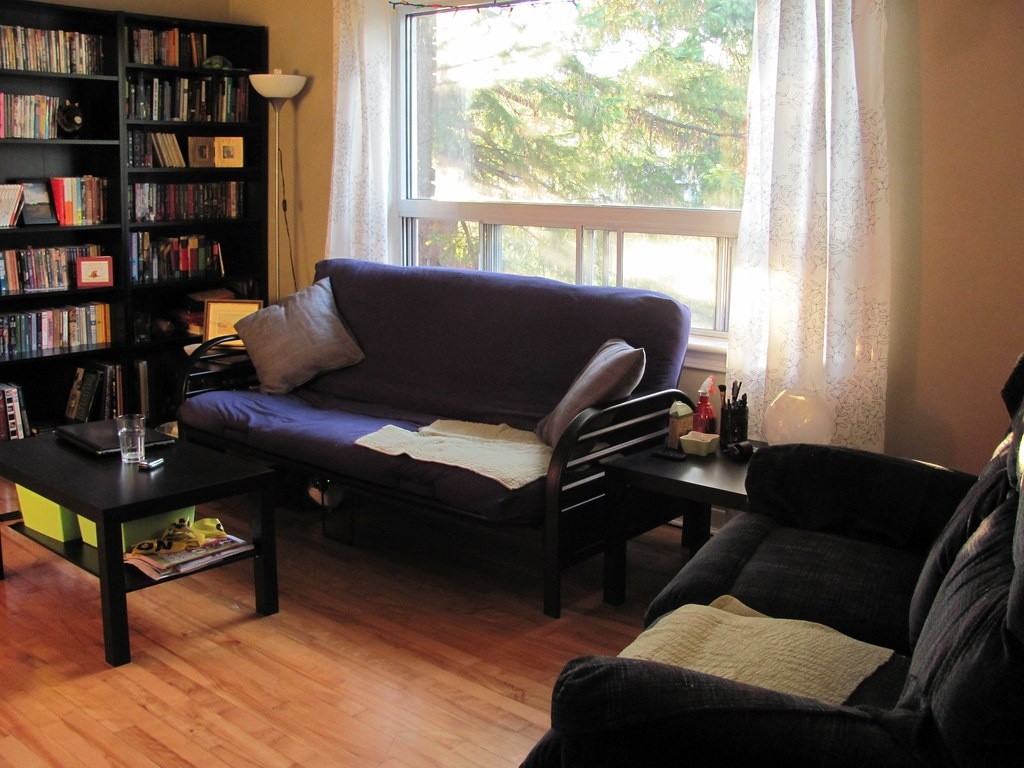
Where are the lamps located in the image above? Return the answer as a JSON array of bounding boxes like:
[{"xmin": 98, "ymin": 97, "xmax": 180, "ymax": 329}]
[{"xmin": 249, "ymin": 69, "xmax": 306, "ymax": 302}]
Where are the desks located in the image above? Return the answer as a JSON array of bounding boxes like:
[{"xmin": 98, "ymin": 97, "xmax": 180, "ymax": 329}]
[
  {"xmin": 0, "ymin": 429, "xmax": 282, "ymax": 667},
  {"xmin": 192, "ymin": 349, "xmax": 249, "ymax": 390},
  {"xmin": 603, "ymin": 439, "xmax": 768, "ymax": 607}
]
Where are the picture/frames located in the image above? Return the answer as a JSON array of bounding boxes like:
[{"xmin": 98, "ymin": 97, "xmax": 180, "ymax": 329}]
[
  {"xmin": 76, "ymin": 256, "xmax": 113, "ymax": 286},
  {"xmin": 12, "ymin": 178, "xmax": 58, "ymax": 224},
  {"xmin": 203, "ymin": 299, "xmax": 263, "ymax": 347}
]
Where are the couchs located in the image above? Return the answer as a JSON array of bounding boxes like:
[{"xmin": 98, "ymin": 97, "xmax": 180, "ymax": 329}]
[
  {"xmin": 520, "ymin": 354, "xmax": 1024, "ymax": 768},
  {"xmin": 174, "ymin": 261, "xmax": 692, "ymax": 619}
]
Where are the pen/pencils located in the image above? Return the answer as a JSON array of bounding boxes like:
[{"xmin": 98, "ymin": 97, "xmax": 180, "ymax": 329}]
[{"xmin": 728, "ymin": 381, "xmax": 748, "ymax": 410}]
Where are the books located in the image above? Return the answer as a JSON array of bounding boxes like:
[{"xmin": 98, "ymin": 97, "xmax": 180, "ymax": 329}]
[
  {"xmin": 0, "ymin": 95, "xmax": 62, "ymax": 142},
  {"xmin": 128, "ymin": 131, "xmax": 188, "ymax": 169},
  {"xmin": 0, "ymin": 244, "xmax": 106, "ymax": 294},
  {"xmin": 127, "ymin": 181, "xmax": 247, "ymax": 222},
  {"xmin": 0, "ymin": 173, "xmax": 112, "ymax": 228},
  {"xmin": 0, "ymin": 302, "xmax": 112, "ymax": 356},
  {"xmin": 130, "ymin": 231, "xmax": 229, "ymax": 283},
  {"xmin": 54, "ymin": 418, "xmax": 174, "ymax": 454},
  {"xmin": 125, "ymin": 73, "xmax": 250, "ymax": 123},
  {"xmin": 124, "ymin": 25, "xmax": 210, "ymax": 68},
  {"xmin": 0, "ymin": 360, "xmax": 149, "ymax": 442},
  {"xmin": 123, "ymin": 533, "xmax": 254, "ymax": 581},
  {"xmin": 0, "ymin": 24, "xmax": 111, "ymax": 76}
]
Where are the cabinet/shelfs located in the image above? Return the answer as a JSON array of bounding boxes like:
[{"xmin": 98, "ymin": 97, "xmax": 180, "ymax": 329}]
[{"xmin": 0, "ymin": 0, "xmax": 269, "ymax": 442}]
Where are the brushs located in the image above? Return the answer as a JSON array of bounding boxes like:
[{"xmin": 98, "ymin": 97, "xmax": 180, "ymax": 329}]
[{"xmin": 718, "ymin": 384, "xmax": 727, "ymax": 406}]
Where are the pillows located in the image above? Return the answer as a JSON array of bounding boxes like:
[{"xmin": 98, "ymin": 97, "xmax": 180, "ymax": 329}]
[
  {"xmin": 235, "ymin": 275, "xmax": 365, "ymax": 394},
  {"xmin": 534, "ymin": 338, "xmax": 646, "ymax": 460}
]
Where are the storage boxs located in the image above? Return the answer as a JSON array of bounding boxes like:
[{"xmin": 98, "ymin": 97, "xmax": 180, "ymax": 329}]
[
  {"xmin": 15, "ymin": 481, "xmax": 195, "ymax": 553},
  {"xmin": 668, "ymin": 401, "xmax": 693, "ymax": 449}
]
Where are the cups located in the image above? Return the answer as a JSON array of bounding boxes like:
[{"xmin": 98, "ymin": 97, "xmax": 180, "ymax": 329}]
[
  {"xmin": 719, "ymin": 405, "xmax": 749, "ymax": 451},
  {"xmin": 116, "ymin": 413, "xmax": 147, "ymax": 464}
]
